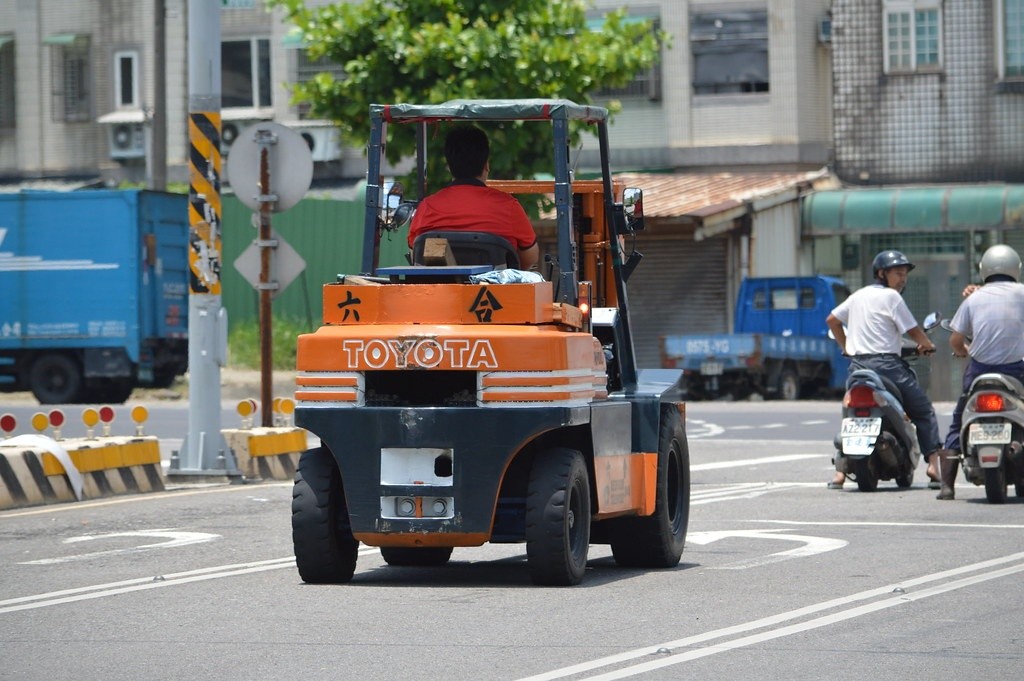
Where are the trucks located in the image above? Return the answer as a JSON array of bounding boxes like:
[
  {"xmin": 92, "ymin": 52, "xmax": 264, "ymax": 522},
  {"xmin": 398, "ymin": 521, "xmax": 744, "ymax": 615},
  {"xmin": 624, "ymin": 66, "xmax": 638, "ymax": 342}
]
[
  {"xmin": 655, "ymin": 275, "xmax": 856, "ymax": 402},
  {"xmin": 0, "ymin": 186, "xmax": 197, "ymax": 409}
]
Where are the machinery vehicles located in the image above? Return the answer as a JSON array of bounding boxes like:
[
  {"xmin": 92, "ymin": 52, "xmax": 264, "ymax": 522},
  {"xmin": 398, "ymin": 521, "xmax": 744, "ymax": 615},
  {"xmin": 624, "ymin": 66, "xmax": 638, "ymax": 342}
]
[{"xmin": 293, "ymin": 98, "xmax": 695, "ymax": 588}]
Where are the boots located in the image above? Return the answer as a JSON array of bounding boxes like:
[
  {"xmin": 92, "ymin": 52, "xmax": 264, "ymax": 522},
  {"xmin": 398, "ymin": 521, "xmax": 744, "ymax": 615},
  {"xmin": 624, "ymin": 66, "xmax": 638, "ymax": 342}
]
[{"xmin": 936, "ymin": 456, "xmax": 959, "ymax": 500}]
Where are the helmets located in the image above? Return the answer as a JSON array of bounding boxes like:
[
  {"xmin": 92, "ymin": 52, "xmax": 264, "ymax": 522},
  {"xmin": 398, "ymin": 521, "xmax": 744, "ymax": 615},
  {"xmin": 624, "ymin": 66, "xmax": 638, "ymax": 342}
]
[
  {"xmin": 979, "ymin": 245, "xmax": 1022, "ymax": 283},
  {"xmin": 873, "ymin": 250, "xmax": 915, "ymax": 278}
]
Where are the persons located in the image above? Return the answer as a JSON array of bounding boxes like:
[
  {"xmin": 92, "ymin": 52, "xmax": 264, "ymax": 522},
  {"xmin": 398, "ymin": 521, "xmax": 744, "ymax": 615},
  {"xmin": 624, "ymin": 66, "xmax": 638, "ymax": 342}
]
[
  {"xmin": 407, "ymin": 124, "xmax": 539, "ymax": 271},
  {"xmin": 936, "ymin": 243, "xmax": 1024, "ymax": 501},
  {"xmin": 826, "ymin": 250, "xmax": 942, "ymax": 489}
]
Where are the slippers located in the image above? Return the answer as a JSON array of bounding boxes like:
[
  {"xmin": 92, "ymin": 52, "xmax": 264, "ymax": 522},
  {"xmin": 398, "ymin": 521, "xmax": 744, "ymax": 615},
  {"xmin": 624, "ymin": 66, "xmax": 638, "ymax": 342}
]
[
  {"xmin": 928, "ymin": 481, "xmax": 941, "ymax": 489},
  {"xmin": 828, "ymin": 480, "xmax": 842, "ymax": 489}
]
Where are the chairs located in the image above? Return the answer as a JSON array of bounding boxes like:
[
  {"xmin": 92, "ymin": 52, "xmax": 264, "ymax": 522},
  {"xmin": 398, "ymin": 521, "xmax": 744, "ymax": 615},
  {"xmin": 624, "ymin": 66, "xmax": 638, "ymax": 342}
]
[{"xmin": 411, "ymin": 231, "xmax": 521, "ymax": 270}]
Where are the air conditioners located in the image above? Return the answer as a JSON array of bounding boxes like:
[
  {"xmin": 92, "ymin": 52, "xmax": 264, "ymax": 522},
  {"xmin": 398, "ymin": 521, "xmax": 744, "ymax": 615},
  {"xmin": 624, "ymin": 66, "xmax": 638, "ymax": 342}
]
[
  {"xmin": 220, "ymin": 120, "xmax": 261, "ymax": 154},
  {"xmin": 300, "ymin": 127, "xmax": 341, "ymax": 161},
  {"xmin": 106, "ymin": 123, "xmax": 144, "ymax": 158},
  {"xmin": 817, "ymin": 15, "xmax": 831, "ymax": 45}
]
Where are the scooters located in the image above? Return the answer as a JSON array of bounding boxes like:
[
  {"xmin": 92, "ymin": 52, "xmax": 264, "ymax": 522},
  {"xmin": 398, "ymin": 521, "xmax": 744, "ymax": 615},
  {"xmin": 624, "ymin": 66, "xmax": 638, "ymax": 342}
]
[
  {"xmin": 936, "ymin": 317, "xmax": 1023, "ymax": 505},
  {"xmin": 830, "ymin": 310, "xmax": 942, "ymax": 490}
]
